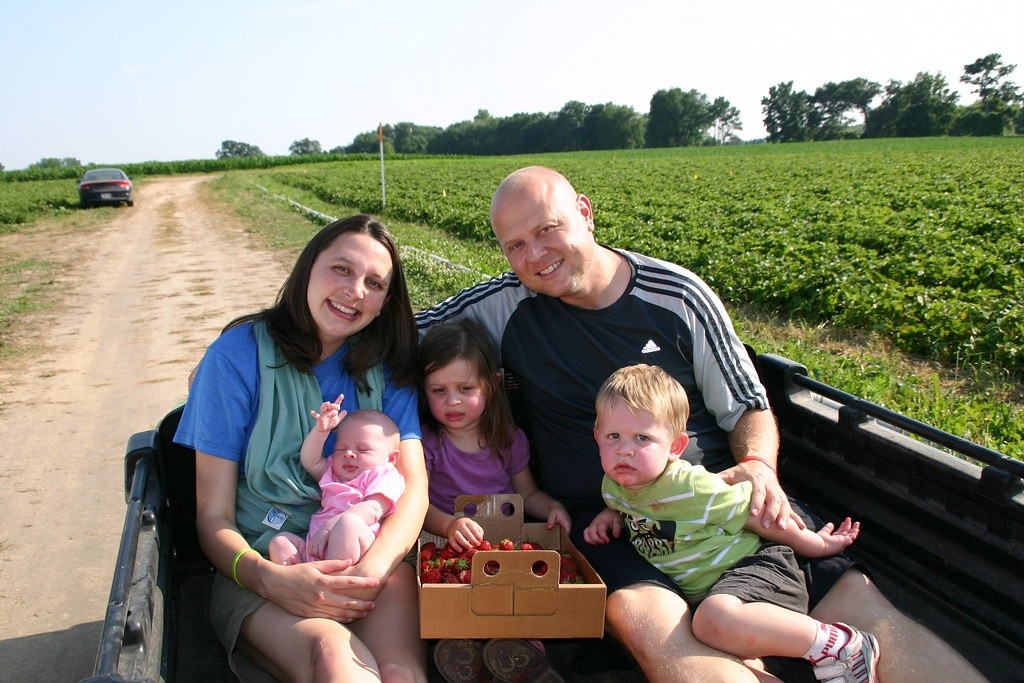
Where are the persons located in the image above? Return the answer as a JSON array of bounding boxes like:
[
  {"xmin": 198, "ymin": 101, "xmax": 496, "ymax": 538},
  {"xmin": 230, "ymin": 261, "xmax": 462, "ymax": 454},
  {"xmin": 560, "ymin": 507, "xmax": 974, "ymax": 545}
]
[
  {"xmin": 417, "ymin": 316, "xmax": 572, "ymax": 682},
  {"xmin": 172, "ymin": 216, "xmax": 431, "ymax": 682},
  {"xmin": 584, "ymin": 363, "xmax": 881, "ymax": 683},
  {"xmin": 187, "ymin": 166, "xmax": 991, "ymax": 683},
  {"xmin": 269, "ymin": 394, "xmax": 407, "ymax": 565}
]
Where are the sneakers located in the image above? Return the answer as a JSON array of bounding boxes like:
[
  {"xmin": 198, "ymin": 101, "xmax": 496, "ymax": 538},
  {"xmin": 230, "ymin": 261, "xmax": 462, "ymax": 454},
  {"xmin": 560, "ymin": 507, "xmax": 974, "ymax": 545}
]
[{"xmin": 811, "ymin": 621, "xmax": 880, "ymax": 683}]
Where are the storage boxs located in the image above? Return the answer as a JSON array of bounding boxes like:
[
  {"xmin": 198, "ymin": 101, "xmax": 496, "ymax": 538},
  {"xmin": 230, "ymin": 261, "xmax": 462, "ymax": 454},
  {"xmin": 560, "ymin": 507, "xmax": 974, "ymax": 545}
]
[{"xmin": 415, "ymin": 494, "xmax": 607, "ymax": 638}]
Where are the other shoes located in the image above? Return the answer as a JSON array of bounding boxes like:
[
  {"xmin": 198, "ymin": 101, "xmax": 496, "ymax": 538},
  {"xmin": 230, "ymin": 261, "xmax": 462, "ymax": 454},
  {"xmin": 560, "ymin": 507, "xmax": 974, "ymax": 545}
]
[{"xmin": 433, "ymin": 639, "xmax": 565, "ymax": 683}]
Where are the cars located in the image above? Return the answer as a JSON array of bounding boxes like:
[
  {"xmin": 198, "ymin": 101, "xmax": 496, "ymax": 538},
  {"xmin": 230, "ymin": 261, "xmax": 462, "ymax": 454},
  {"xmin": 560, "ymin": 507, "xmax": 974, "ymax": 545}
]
[{"xmin": 75, "ymin": 168, "xmax": 134, "ymax": 208}]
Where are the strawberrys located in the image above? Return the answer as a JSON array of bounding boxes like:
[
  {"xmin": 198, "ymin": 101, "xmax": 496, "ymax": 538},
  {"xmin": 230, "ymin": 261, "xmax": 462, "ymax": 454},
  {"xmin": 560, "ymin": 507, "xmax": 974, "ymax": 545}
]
[{"xmin": 420, "ymin": 537, "xmax": 584, "ymax": 585}]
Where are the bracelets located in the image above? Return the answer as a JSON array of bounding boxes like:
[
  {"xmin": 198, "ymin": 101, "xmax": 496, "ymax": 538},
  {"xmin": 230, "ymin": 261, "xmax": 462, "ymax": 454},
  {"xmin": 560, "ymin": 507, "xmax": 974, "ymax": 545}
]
[
  {"xmin": 231, "ymin": 547, "xmax": 262, "ymax": 590},
  {"xmin": 737, "ymin": 456, "xmax": 779, "ymax": 480}
]
